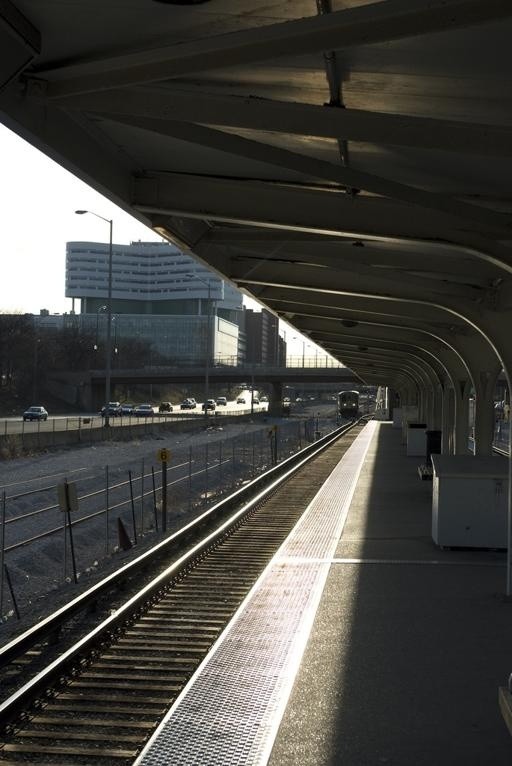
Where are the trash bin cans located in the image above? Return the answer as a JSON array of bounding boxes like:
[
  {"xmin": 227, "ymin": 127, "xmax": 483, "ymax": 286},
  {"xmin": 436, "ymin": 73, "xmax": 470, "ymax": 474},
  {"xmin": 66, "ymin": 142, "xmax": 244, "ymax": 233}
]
[{"xmin": 425, "ymin": 431, "xmax": 442, "ymax": 467}]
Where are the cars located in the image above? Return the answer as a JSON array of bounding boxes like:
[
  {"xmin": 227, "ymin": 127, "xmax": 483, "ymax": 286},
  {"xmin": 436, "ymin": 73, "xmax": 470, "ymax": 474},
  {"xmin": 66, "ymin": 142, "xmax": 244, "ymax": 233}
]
[
  {"xmin": 237, "ymin": 386, "xmax": 268, "ymax": 405},
  {"xmin": 100, "ymin": 396, "xmax": 197, "ymax": 417},
  {"xmin": 23, "ymin": 406, "xmax": 49, "ymax": 421},
  {"xmin": 284, "ymin": 397, "xmax": 316, "ymax": 402}
]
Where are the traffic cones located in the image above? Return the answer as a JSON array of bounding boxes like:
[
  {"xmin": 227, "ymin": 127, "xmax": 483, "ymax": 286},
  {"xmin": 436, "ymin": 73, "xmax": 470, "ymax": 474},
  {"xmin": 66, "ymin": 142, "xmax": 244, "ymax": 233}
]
[{"xmin": 115, "ymin": 517, "xmax": 134, "ymax": 551}]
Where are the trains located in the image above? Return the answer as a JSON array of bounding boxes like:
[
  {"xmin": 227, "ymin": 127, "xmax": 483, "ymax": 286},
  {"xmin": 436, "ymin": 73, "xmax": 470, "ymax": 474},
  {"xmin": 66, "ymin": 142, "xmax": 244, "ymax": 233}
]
[{"xmin": 338, "ymin": 390, "xmax": 374, "ymax": 418}]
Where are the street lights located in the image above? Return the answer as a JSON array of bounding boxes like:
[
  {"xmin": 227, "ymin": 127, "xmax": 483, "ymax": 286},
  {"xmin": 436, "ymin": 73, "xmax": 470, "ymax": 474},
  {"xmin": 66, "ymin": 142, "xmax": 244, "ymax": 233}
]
[
  {"xmin": 111, "ymin": 312, "xmax": 121, "ymax": 370},
  {"xmin": 219, "ymin": 352, "xmax": 222, "ymax": 368},
  {"xmin": 94, "ymin": 306, "xmax": 107, "ymax": 370},
  {"xmin": 75, "ymin": 209, "xmax": 113, "ymax": 428},
  {"xmin": 272, "ymin": 325, "xmax": 318, "ymax": 368},
  {"xmin": 186, "ymin": 273, "xmax": 211, "ymax": 416},
  {"xmin": 150, "ymin": 335, "xmax": 169, "ymax": 398}
]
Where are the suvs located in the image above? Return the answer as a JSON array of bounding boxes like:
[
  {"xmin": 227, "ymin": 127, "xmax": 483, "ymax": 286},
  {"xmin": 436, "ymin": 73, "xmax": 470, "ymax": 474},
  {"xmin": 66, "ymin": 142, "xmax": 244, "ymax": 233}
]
[
  {"xmin": 217, "ymin": 397, "xmax": 226, "ymax": 406},
  {"xmin": 202, "ymin": 399, "xmax": 216, "ymax": 411}
]
